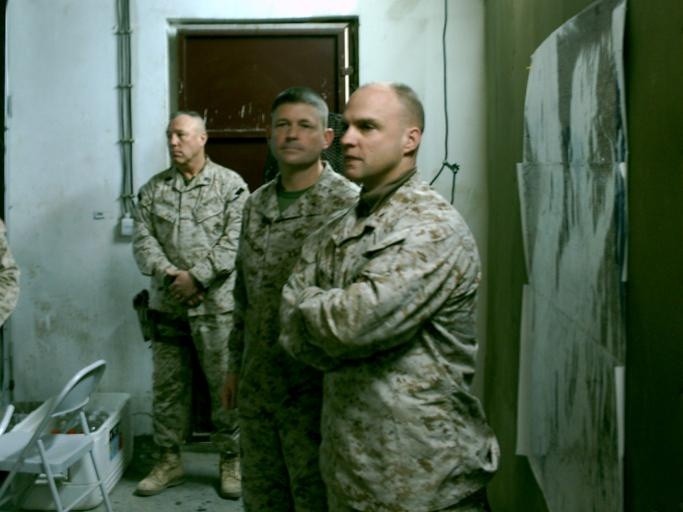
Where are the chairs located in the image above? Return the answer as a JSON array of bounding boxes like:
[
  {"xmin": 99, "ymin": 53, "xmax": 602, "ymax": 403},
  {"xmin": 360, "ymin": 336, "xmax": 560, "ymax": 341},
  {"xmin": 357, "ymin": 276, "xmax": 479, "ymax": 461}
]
[{"xmin": 1, "ymin": 359, "xmax": 114, "ymax": 512}]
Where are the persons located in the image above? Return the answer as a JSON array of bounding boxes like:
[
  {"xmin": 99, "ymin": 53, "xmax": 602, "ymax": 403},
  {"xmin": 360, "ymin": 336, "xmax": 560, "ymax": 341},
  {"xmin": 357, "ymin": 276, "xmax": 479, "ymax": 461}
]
[
  {"xmin": 131, "ymin": 110, "xmax": 250, "ymax": 500},
  {"xmin": 0, "ymin": 216, "xmax": 20, "ymax": 328},
  {"xmin": 221, "ymin": 84, "xmax": 360, "ymax": 512},
  {"xmin": 277, "ymin": 81, "xmax": 501, "ymax": 512}
]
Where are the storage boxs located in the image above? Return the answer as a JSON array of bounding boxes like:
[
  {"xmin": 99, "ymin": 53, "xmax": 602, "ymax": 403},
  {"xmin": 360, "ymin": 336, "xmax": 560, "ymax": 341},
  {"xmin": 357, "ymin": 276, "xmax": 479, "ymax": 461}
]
[{"xmin": 10, "ymin": 392, "xmax": 133, "ymax": 510}]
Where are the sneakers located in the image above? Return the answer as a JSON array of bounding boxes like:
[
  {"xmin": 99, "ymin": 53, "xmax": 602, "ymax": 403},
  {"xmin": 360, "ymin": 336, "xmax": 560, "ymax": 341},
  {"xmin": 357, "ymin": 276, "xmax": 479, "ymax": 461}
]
[
  {"xmin": 135, "ymin": 450, "xmax": 186, "ymax": 498},
  {"xmin": 216, "ymin": 452, "xmax": 243, "ymax": 500}
]
